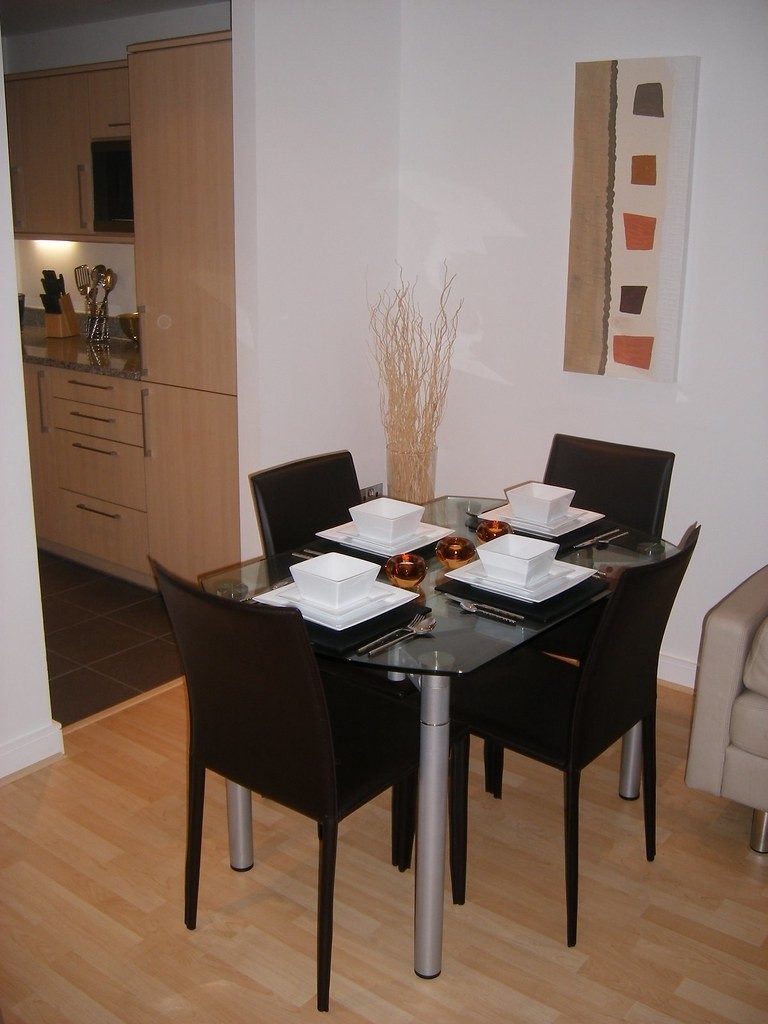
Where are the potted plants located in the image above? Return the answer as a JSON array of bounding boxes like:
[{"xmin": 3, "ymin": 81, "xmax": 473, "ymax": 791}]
[{"xmin": 364, "ymin": 260, "xmax": 464, "ymax": 526}]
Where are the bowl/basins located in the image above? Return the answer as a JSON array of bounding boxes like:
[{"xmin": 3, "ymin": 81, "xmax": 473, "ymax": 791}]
[
  {"xmin": 476, "ymin": 533, "xmax": 560, "ymax": 586},
  {"xmin": 117, "ymin": 312, "xmax": 139, "ymax": 342},
  {"xmin": 289, "ymin": 552, "xmax": 381, "ymax": 610},
  {"xmin": 349, "ymin": 497, "xmax": 425, "ymax": 544},
  {"xmin": 506, "ymin": 482, "xmax": 576, "ymax": 523}
]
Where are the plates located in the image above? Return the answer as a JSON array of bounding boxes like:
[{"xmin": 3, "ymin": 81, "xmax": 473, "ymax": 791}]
[
  {"xmin": 315, "ymin": 519, "xmax": 456, "ymax": 558},
  {"xmin": 476, "ymin": 503, "xmax": 606, "ymax": 537},
  {"xmin": 249, "ymin": 580, "xmax": 421, "ymax": 630},
  {"xmin": 444, "ymin": 559, "xmax": 598, "ymax": 603}
]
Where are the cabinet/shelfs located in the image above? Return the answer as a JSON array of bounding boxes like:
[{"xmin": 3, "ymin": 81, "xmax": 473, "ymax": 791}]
[
  {"xmin": 51, "ymin": 366, "xmax": 149, "ymax": 592},
  {"xmin": 140, "ymin": 381, "xmax": 242, "ymax": 604},
  {"xmin": 4, "ymin": 59, "xmax": 135, "ymax": 244},
  {"xmin": 24, "ymin": 362, "xmax": 51, "ymax": 554},
  {"xmin": 127, "ymin": 29, "xmax": 237, "ymax": 396}
]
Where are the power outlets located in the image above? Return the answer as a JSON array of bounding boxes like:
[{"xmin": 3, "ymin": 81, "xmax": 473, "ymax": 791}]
[{"xmin": 360, "ymin": 482, "xmax": 383, "ymax": 504}]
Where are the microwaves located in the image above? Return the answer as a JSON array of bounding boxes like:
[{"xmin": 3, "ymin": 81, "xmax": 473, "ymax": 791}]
[{"xmin": 92, "ymin": 138, "xmax": 135, "ymax": 234}]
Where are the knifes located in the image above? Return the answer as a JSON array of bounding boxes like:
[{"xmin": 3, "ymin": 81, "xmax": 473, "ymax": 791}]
[
  {"xmin": 573, "ymin": 528, "xmax": 619, "ymax": 548},
  {"xmin": 38, "ymin": 270, "xmax": 65, "ymax": 315},
  {"xmin": 445, "ymin": 593, "xmax": 525, "ymax": 620}
]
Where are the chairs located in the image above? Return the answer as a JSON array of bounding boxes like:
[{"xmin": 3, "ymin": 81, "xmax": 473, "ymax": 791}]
[
  {"xmin": 542, "ymin": 433, "xmax": 675, "ymax": 538},
  {"xmin": 146, "ymin": 554, "xmax": 420, "ymax": 1012},
  {"xmin": 403, "ymin": 526, "xmax": 702, "ymax": 948},
  {"xmin": 248, "ymin": 449, "xmax": 415, "ymax": 694}
]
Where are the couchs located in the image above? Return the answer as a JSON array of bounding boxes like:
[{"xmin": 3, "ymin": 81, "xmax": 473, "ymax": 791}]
[{"xmin": 683, "ymin": 562, "xmax": 768, "ymax": 853}]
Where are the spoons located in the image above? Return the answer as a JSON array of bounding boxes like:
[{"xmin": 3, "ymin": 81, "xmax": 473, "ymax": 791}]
[
  {"xmin": 460, "ymin": 602, "xmax": 516, "ymax": 625},
  {"xmin": 367, "ymin": 617, "xmax": 437, "ymax": 657},
  {"xmin": 598, "ymin": 532, "xmax": 629, "ymax": 544}
]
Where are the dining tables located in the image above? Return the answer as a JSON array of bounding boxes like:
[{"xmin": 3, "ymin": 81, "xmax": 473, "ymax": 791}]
[{"xmin": 198, "ymin": 496, "xmax": 681, "ymax": 979}]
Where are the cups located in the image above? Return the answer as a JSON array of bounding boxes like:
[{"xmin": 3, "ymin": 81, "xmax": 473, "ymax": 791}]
[
  {"xmin": 435, "ymin": 536, "xmax": 476, "ymax": 570},
  {"xmin": 384, "ymin": 553, "xmax": 427, "ymax": 588},
  {"xmin": 477, "ymin": 521, "xmax": 513, "ymax": 544}
]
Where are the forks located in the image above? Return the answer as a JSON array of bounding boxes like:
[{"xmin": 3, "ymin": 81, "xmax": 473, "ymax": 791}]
[{"xmin": 357, "ymin": 614, "xmax": 425, "ymax": 654}]
